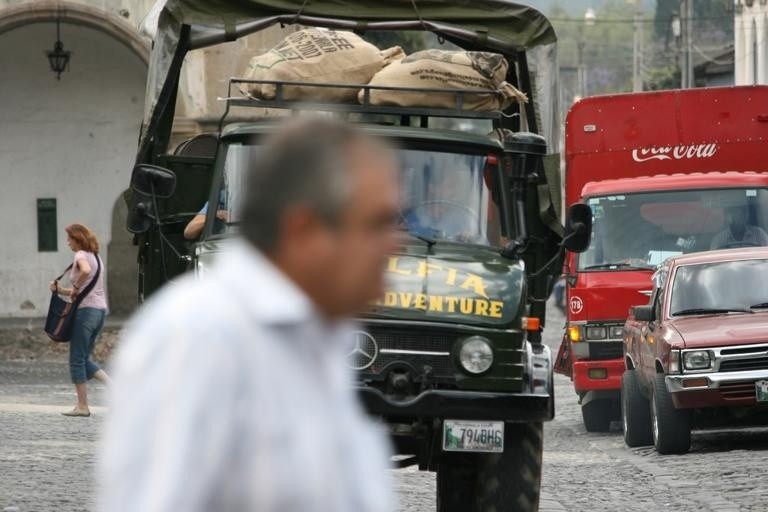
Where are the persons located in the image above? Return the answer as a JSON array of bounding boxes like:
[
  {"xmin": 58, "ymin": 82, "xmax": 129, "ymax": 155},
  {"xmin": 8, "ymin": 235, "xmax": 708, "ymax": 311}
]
[
  {"xmin": 48, "ymin": 223, "xmax": 112, "ymax": 417},
  {"xmin": 396, "ymin": 159, "xmax": 474, "ymax": 245},
  {"xmin": 87, "ymin": 114, "xmax": 404, "ymax": 512},
  {"xmin": 709, "ymin": 200, "xmax": 768, "ymax": 252},
  {"xmin": 184, "ymin": 188, "xmax": 229, "ymax": 242}
]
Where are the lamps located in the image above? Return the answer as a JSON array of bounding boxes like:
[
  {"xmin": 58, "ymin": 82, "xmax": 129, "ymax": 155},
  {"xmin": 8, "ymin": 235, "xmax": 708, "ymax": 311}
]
[{"xmin": 47, "ymin": 19, "xmax": 71, "ymax": 81}]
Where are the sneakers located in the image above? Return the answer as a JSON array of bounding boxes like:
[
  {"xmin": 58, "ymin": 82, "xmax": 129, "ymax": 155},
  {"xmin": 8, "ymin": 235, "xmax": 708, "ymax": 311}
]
[{"xmin": 60, "ymin": 406, "xmax": 90, "ymax": 416}]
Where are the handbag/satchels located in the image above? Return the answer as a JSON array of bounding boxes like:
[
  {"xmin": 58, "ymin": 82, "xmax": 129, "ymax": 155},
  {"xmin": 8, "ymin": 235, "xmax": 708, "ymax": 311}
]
[{"xmin": 45, "ymin": 293, "xmax": 78, "ymax": 342}]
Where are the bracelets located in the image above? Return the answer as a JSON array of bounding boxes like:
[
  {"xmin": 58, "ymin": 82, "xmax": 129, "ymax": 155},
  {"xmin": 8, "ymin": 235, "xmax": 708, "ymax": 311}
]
[{"xmin": 73, "ymin": 284, "xmax": 80, "ymax": 290}]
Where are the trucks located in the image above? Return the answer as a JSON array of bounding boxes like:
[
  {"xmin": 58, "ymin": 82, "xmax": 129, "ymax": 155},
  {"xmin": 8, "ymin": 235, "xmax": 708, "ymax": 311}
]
[
  {"xmin": 123, "ymin": 1, "xmax": 595, "ymax": 511},
  {"xmin": 556, "ymin": 77, "xmax": 768, "ymax": 434}
]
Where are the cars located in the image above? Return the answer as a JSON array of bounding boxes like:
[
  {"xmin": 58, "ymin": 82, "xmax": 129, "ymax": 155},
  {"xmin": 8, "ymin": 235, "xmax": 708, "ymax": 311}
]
[{"xmin": 619, "ymin": 239, "xmax": 768, "ymax": 457}]
